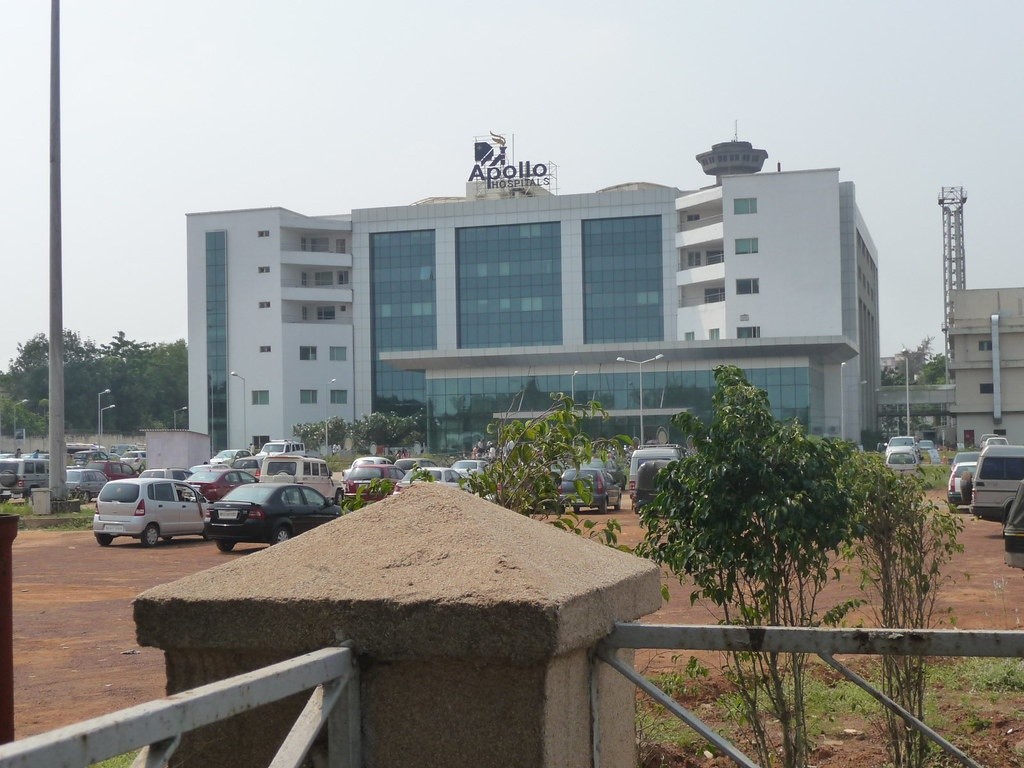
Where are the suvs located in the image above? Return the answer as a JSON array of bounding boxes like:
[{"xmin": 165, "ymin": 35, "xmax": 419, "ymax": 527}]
[{"xmin": 0, "ymin": 453, "xmax": 50, "ymax": 494}]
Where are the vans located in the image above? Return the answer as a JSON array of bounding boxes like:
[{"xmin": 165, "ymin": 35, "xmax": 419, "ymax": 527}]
[
  {"xmin": 255, "ymin": 440, "xmax": 333, "ymax": 488},
  {"xmin": 67, "ymin": 443, "xmax": 147, "ymax": 504},
  {"xmin": 629, "ymin": 444, "xmax": 689, "ymax": 502},
  {"xmin": 970, "ymin": 444, "xmax": 1024, "ymax": 525},
  {"xmin": 92, "ymin": 467, "xmax": 212, "ymax": 548}
]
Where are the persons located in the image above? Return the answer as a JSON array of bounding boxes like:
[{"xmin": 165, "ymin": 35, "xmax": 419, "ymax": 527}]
[
  {"xmin": 32, "ymin": 449, "xmax": 39, "ymax": 458},
  {"xmin": 15, "ymin": 448, "xmax": 22, "ymax": 458},
  {"xmin": 249, "ymin": 443, "xmax": 254, "ymax": 454},
  {"xmin": 471, "ymin": 438, "xmax": 496, "ymax": 462},
  {"xmin": 396, "ymin": 449, "xmax": 409, "ymax": 460},
  {"xmin": 333, "ymin": 442, "xmax": 339, "ymax": 454}
]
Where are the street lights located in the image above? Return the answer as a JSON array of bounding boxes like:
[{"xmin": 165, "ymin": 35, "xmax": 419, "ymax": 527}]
[
  {"xmin": 13, "ymin": 398, "xmax": 29, "ymax": 451},
  {"xmin": 98, "ymin": 388, "xmax": 116, "ymax": 451},
  {"xmin": 325, "ymin": 378, "xmax": 336, "ymax": 460},
  {"xmin": 230, "ymin": 372, "xmax": 247, "ymax": 448},
  {"xmin": 571, "ymin": 370, "xmax": 579, "ymax": 410},
  {"xmin": 173, "ymin": 407, "xmax": 188, "ymax": 430},
  {"xmin": 616, "ymin": 354, "xmax": 665, "ymax": 446}
]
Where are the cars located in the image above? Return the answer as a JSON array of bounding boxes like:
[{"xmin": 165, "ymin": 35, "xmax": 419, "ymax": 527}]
[
  {"xmin": 881, "ymin": 436, "xmax": 934, "ymax": 473},
  {"xmin": 1001, "ymin": 478, "xmax": 1024, "ymax": 571},
  {"xmin": 202, "ymin": 482, "xmax": 344, "ymax": 553},
  {"xmin": 181, "ymin": 450, "xmax": 260, "ymax": 502},
  {"xmin": 632, "ymin": 459, "xmax": 676, "ymax": 514},
  {"xmin": 947, "ymin": 432, "xmax": 1008, "ymax": 506},
  {"xmin": 339, "ymin": 455, "xmax": 628, "ymax": 513}
]
